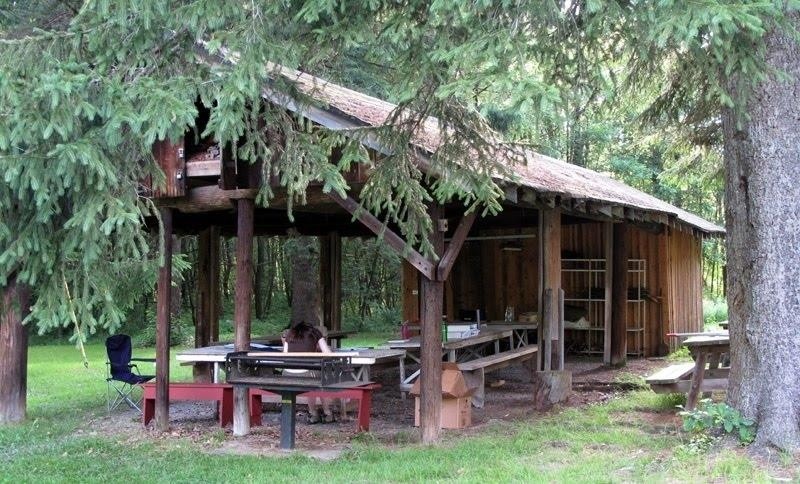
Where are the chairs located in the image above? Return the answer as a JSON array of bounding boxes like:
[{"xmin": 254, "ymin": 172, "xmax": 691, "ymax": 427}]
[{"xmin": 104, "ymin": 332, "xmax": 158, "ymax": 416}]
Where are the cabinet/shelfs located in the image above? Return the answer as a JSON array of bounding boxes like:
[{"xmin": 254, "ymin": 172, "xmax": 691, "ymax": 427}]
[{"xmin": 560, "ymin": 257, "xmax": 649, "ymax": 356}]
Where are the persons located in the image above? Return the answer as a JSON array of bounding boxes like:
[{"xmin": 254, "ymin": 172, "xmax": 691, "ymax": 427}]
[{"xmin": 283, "ymin": 320, "xmax": 332, "ymax": 374}]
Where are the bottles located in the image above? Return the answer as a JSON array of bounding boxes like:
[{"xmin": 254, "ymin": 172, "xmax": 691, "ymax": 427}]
[
  {"xmin": 442, "ymin": 320, "xmax": 449, "ymax": 343},
  {"xmin": 401, "ymin": 322, "xmax": 410, "ymax": 339},
  {"xmin": 505, "ymin": 305, "xmax": 512, "ymax": 322}
]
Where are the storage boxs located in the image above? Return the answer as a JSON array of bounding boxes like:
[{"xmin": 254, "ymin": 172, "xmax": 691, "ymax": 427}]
[
  {"xmin": 414, "ymin": 396, "xmax": 471, "ymax": 429},
  {"xmin": 410, "ymin": 361, "xmax": 469, "ymax": 396}
]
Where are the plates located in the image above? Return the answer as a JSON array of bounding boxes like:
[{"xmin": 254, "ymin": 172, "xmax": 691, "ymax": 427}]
[{"xmin": 389, "ymin": 338, "xmax": 413, "ymax": 343}]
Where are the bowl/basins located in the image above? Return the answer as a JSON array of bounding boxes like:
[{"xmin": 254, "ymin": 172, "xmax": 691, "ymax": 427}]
[{"xmin": 458, "ymin": 309, "xmax": 477, "ymax": 322}]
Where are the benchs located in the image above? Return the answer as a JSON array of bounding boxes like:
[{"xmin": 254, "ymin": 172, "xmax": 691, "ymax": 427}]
[
  {"xmin": 457, "ymin": 343, "xmax": 539, "ymax": 409},
  {"xmin": 246, "ymin": 382, "xmax": 383, "ymax": 436},
  {"xmin": 141, "ymin": 378, "xmax": 236, "ymax": 428},
  {"xmin": 644, "ymin": 363, "xmax": 696, "ymax": 394}
]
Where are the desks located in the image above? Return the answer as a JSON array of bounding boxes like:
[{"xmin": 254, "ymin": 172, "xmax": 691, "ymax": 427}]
[
  {"xmin": 682, "ymin": 330, "xmax": 730, "ymax": 411},
  {"xmin": 493, "ymin": 320, "xmax": 539, "ymax": 349},
  {"xmin": 175, "ymin": 330, "xmax": 410, "ymax": 420},
  {"xmin": 390, "ymin": 325, "xmax": 514, "ymax": 403}
]
